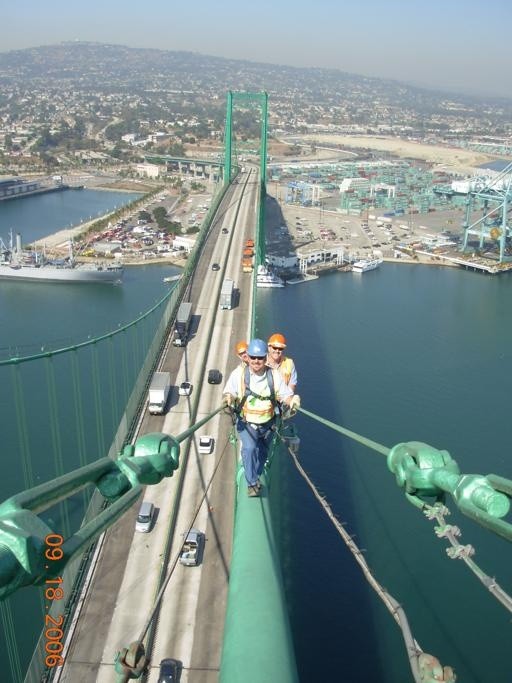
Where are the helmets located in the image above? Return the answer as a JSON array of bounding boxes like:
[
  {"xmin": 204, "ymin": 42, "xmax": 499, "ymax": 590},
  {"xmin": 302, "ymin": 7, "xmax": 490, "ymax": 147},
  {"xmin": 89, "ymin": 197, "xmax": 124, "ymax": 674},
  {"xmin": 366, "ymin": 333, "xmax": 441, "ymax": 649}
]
[
  {"xmin": 268, "ymin": 332, "xmax": 287, "ymax": 348},
  {"xmin": 246, "ymin": 338, "xmax": 269, "ymax": 357},
  {"xmin": 236, "ymin": 342, "xmax": 248, "ymax": 354}
]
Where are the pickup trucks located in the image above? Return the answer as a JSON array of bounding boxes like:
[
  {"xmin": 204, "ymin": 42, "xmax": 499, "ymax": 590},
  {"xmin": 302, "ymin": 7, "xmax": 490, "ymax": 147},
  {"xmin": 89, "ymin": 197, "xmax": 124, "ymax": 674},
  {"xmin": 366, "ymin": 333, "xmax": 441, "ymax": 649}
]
[{"xmin": 179, "ymin": 528, "xmax": 202, "ymax": 566}]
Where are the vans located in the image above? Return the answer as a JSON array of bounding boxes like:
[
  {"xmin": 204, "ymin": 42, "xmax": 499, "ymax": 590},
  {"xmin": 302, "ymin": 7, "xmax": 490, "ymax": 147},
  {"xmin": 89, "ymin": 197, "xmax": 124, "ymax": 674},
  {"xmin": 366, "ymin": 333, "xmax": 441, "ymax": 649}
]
[{"xmin": 134, "ymin": 501, "xmax": 155, "ymax": 531}]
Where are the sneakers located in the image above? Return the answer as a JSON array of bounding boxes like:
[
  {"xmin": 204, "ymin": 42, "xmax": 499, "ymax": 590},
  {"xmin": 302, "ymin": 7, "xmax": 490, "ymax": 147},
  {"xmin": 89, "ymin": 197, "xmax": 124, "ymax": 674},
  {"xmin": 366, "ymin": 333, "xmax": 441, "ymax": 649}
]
[{"xmin": 248, "ymin": 485, "xmax": 260, "ymax": 496}]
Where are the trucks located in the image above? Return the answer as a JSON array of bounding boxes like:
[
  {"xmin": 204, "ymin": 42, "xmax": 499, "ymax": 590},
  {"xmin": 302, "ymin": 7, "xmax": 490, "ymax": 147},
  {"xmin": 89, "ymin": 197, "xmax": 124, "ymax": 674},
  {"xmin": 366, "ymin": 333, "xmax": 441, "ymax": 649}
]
[
  {"xmin": 242, "ymin": 239, "xmax": 255, "ymax": 272},
  {"xmin": 171, "ymin": 302, "xmax": 194, "ymax": 347},
  {"xmin": 146, "ymin": 369, "xmax": 171, "ymax": 416},
  {"xmin": 218, "ymin": 277, "xmax": 235, "ymax": 309}
]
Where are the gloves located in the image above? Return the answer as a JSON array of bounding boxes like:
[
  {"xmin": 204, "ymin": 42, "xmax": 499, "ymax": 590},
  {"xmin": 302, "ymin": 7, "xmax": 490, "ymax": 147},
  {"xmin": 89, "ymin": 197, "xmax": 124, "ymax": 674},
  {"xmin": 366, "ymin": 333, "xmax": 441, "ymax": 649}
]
[
  {"xmin": 290, "ymin": 394, "xmax": 300, "ymax": 411},
  {"xmin": 222, "ymin": 393, "xmax": 235, "ymax": 414}
]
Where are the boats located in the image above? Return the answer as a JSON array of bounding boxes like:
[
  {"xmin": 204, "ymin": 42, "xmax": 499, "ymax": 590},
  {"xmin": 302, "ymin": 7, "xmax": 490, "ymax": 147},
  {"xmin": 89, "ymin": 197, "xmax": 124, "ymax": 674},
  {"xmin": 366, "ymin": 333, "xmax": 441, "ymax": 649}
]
[
  {"xmin": 257, "ymin": 249, "xmax": 386, "ymax": 286},
  {"xmin": 0, "ymin": 227, "xmax": 126, "ymax": 280}
]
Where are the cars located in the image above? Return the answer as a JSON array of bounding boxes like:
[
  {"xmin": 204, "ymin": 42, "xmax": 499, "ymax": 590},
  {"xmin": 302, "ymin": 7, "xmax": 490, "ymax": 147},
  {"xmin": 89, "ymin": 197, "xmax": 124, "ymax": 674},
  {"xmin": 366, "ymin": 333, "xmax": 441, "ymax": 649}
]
[
  {"xmin": 187, "ymin": 197, "xmax": 212, "ymax": 230},
  {"xmin": 199, "ymin": 435, "xmax": 213, "ymax": 454},
  {"xmin": 268, "ymin": 214, "xmax": 409, "ymax": 248},
  {"xmin": 72, "ymin": 192, "xmax": 185, "ymax": 260},
  {"xmin": 178, "ymin": 382, "xmax": 192, "ymax": 394},
  {"xmin": 208, "ymin": 367, "xmax": 220, "ymax": 384},
  {"xmin": 156, "ymin": 658, "xmax": 178, "ymax": 682},
  {"xmin": 211, "ymin": 263, "xmax": 219, "ymax": 270},
  {"xmin": 221, "ymin": 228, "xmax": 228, "ymax": 233}
]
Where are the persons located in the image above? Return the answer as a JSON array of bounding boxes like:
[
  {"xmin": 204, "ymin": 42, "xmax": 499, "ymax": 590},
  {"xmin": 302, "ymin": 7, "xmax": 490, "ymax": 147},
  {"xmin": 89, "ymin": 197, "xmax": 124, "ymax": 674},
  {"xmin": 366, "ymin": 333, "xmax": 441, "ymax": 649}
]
[
  {"xmin": 263, "ymin": 332, "xmax": 299, "ymax": 421},
  {"xmin": 220, "ymin": 338, "xmax": 302, "ymax": 498},
  {"xmin": 235, "ymin": 341, "xmax": 252, "ymax": 367}
]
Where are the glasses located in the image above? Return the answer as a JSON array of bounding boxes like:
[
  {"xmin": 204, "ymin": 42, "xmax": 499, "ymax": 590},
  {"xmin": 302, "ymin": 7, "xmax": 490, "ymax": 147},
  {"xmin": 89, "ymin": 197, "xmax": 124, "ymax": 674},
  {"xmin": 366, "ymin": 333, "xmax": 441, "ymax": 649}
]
[
  {"xmin": 273, "ymin": 347, "xmax": 284, "ymax": 350},
  {"xmin": 250, "ymin": 356, "xmax": 264, "ymax": 359}
]
